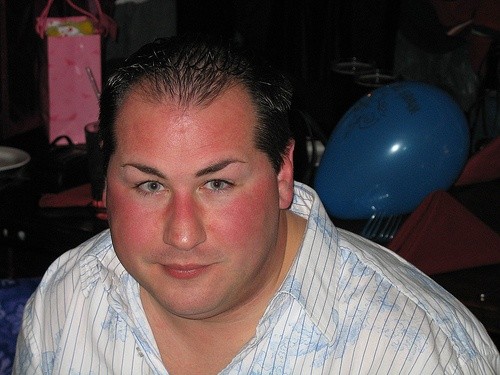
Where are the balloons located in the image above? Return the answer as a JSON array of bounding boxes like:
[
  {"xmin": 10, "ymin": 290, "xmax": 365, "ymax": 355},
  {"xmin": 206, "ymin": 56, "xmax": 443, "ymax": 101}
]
[{"xmin": 311, "ymin": 82, "xmax": 471, "ymax": 221}]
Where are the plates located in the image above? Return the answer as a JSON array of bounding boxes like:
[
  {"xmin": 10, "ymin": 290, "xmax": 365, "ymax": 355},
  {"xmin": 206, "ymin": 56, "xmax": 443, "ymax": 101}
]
[{"xmin": 0, "ymin": 146, "xmax": 30, "ymax": 172}]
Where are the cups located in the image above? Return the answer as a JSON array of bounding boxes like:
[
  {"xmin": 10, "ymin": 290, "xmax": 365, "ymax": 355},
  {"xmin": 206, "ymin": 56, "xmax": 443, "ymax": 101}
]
[{"xmin": 84, "ymin": 121, "xmax": 106, "ymax": 208}]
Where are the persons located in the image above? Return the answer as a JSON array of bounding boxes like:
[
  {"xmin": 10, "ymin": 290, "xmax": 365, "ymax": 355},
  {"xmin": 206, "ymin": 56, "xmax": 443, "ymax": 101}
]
[{"xmin": 12, "ymin": 28, "xmax": 500, "ymax": 375}]
